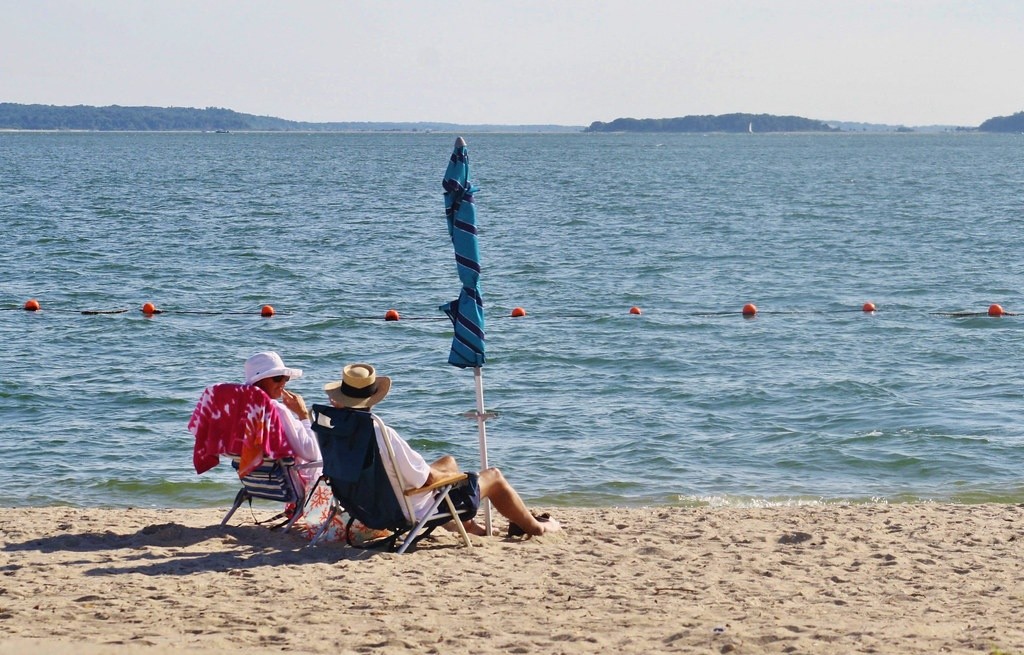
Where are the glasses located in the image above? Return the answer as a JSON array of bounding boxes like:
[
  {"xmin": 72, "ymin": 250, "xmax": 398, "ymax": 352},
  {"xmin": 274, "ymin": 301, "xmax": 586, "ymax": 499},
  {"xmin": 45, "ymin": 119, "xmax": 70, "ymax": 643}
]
[{"xmin": 271, "ymin": 376, "xmax": 290, "ymax": 382}]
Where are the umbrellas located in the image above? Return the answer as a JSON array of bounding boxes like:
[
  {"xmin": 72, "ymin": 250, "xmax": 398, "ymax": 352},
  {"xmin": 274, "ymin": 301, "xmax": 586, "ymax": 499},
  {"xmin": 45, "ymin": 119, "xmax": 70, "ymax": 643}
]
[{"xmin": 439, "ymin": 136, "xmax": 492, "ymax": 536}]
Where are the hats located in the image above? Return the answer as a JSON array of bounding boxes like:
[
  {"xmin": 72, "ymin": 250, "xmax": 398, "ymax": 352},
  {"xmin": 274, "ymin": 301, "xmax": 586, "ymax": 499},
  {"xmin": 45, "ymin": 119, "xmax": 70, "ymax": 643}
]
[
  {"xmin": 324, "ymin": 364, "xmax": 392, "ymax": 408},
  {"xmin": 242, "ymin": 351, "xmax": 303, "ymax": 387}
]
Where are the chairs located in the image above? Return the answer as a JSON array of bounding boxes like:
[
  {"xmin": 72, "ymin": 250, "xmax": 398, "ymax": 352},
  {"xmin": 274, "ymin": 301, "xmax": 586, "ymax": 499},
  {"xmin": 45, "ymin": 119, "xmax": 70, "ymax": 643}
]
[{"xmin": 186, "ymin": 384, "xmax": 478, "ymax": 556}]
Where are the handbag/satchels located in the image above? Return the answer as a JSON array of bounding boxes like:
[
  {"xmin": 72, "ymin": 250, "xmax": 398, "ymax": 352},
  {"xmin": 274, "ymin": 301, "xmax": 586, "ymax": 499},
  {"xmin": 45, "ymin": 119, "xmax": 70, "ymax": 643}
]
[{"xmin": 508, "ymin": 512, "xmax": 560, "ymax": 536}]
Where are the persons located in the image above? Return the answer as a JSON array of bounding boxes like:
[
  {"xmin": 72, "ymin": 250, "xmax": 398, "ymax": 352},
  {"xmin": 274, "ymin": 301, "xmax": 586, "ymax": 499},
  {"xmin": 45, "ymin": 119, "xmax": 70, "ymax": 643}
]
[
  {"xmin": 322, "ymin": 363, "xmax": 561, "ymax": 539},
  {"xmin": 244, "ymin": 351, "xmax": 323, "ymax": 481}
]
